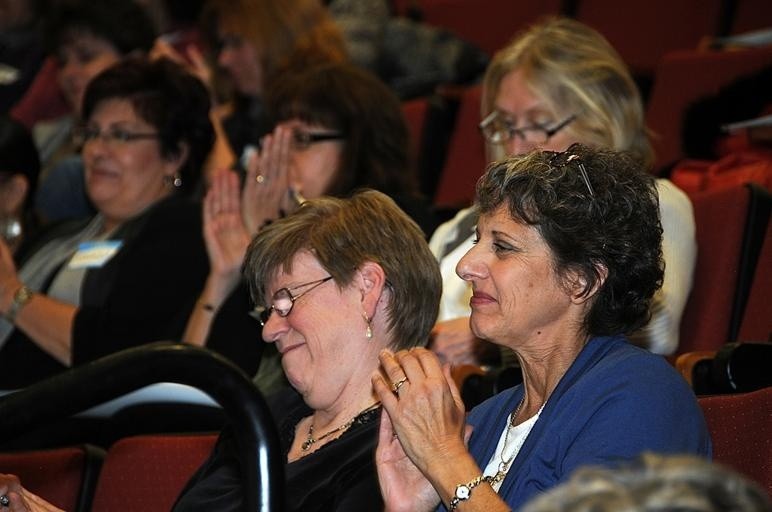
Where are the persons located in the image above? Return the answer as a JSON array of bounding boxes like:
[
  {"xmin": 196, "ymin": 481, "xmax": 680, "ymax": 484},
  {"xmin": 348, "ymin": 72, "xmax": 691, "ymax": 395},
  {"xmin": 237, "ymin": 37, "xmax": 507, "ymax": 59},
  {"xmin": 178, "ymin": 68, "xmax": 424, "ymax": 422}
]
[{"xmin": 0, "ymin": 0, "xmax": 772, "ymax": 512}]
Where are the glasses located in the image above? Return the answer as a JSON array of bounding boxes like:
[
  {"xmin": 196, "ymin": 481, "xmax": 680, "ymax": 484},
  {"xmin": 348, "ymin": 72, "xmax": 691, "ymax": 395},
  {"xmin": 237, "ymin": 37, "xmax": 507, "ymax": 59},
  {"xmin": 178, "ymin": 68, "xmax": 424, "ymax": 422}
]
[
  {"xmin": 72, "ymin": 127, "xmax": 159, "ymax": 146},
  {"xmin": 292, "ymin": 130, "xmax": 344, "ymax": 149},
  {"xmin": 550, "ymin": 150, "xmax": 595, "ymax": 201},
  {"xmin": 477, "ymin": 110, "xmax": 576, "ymax": 145},
  {"xmin": 260, "ymin": 268, "xmax": 396, "ymax": 326}
]
